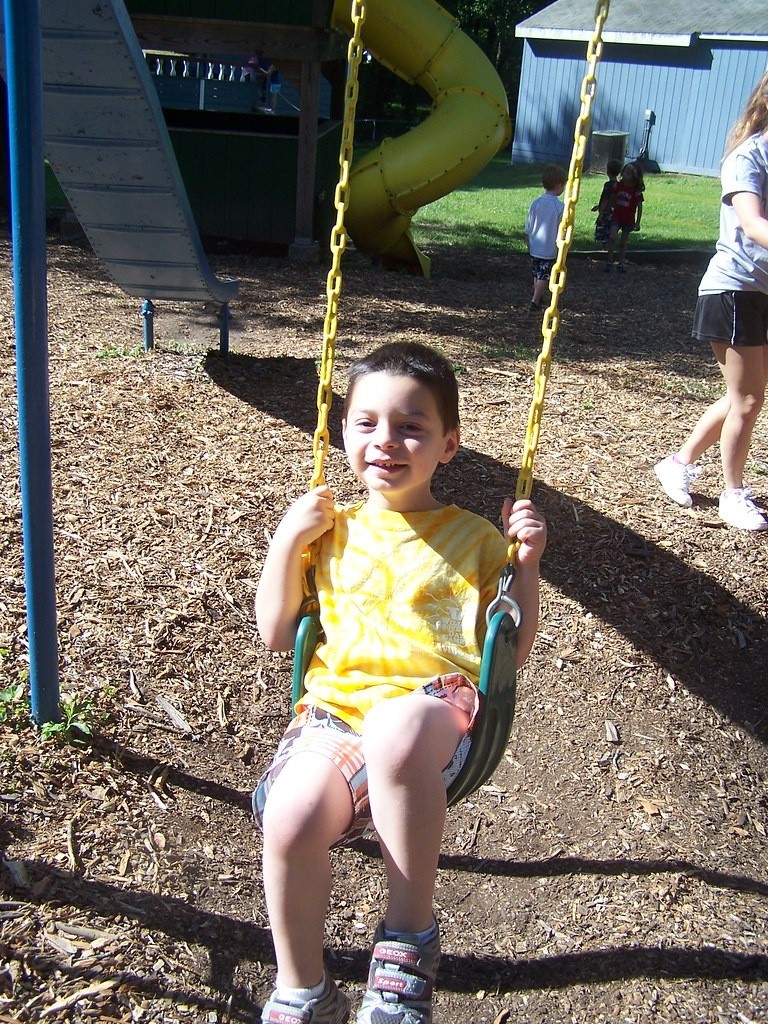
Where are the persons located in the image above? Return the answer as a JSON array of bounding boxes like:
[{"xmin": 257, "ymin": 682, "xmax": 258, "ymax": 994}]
[
  {"xmin": 655, "ymin": 71, "xmax": 767, "ymax": 531},
  {"xmin": 244, "ymin": 57, "xmax": 259, "ymax": 81},
  {"xmin": 591, "ymin": 159, "xmax": 645, "ymax": 273},
  {"xmin": 270, "ymin": 70, "xmax": 281, "ymax": 111},
  {"xmin": 523, "ymin": 165, "xmax": 567, "ymax": 312},
  {"xmin": 252, "ymin": 345, "xmax": 547, "ymax": 1023}
]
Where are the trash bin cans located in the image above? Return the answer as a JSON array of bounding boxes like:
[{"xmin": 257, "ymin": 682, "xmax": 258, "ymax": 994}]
[{"xmin": 589, "ymin": 129, "xmax": 631, "ymax": 178}]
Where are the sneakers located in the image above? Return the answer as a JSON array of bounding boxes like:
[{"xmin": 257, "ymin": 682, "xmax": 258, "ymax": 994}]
[
  {"xmin": 654, "ymin": 454, "xmax": 693, "ymax": 508},
  {"xmin": 529, "ymin": 302, "xmax": 545, "ymax": 311},
  {"xmin": 718, "ymin": 489, "xmax": 767, "ymax": 530},
  {"xmin": 359, "ymin": 910, "xmax": 440, "ymax": 1024},
  {"xmin": 261, "ymin": 964, "xmax": 351, "ymax": 1024}
]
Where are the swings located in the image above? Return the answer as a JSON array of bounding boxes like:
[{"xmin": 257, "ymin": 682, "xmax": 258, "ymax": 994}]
[{"xmin": 288, "ymin": 1, "xmax": 615, "ymax": 808}]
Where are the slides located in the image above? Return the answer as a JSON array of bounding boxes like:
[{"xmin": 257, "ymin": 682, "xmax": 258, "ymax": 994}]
[{"xmin": 327, "ymin": 1, "xmax": 511, "ymax": 278}]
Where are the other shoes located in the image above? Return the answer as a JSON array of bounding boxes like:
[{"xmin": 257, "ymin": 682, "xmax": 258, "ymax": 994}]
[
  {"xmin": 616, "ymin": 263, "xmax": 626, "ymax": 272},
  {"xmin": 603, "ymin": 258, "xmax": 616, "ymax": 272}
]
